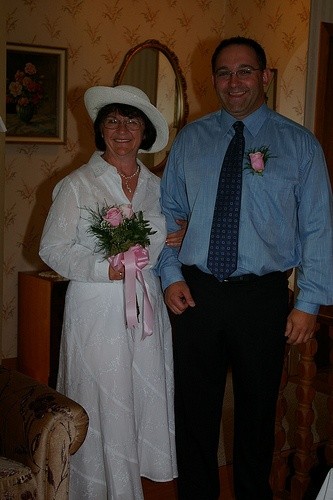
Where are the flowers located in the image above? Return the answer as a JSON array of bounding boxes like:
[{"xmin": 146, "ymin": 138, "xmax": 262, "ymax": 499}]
[
  {"xmin": 78, "ymin": 203, "xmax": 155, "ymax": 262},
  {"xmin": 242, "ymin": 145, "xmax": 280, "ymax": 176}
]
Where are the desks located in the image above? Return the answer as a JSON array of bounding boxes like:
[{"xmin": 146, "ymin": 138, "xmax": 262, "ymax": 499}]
[{"xmin": 18, "ymin": 270, "xmax": 71, "ymax": 387}]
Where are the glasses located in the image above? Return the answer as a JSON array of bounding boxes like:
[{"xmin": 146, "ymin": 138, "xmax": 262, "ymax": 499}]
[
  {"xmin": 212, "ymin": 68, "xmax": 263, "ymax": 80},
  {"xmin": 103, "ymin": 116, "xmax": 144, "ymax": 131}
]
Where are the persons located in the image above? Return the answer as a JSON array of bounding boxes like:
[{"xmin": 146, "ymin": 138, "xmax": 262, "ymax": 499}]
[
  {"xmin": 152, "ymin": 37, "xmax": 333, "ymax": 500},
  {"xmin": 39, "ymin": 85, "xmax": 188, "ymax": 500}
]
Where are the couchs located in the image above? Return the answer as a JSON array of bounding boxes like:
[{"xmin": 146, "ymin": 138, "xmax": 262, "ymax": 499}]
[{"xmin": 0, "ymin": 367, "xmax": 89, "ymax": 500}]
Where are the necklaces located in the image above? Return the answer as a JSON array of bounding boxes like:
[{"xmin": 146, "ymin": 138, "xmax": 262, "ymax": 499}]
[{"xmin": 118, "ymin": 166, "xmax": 140, "ymax": 193}]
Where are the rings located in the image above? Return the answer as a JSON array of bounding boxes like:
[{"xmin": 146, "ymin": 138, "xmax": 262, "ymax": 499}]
[{"xmin": 120, "ymin": 272, "xmax": 122, "ymax": 276}]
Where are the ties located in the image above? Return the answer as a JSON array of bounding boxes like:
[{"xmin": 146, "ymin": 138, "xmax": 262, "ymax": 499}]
[{"xmin": 206, "ymin": 121, "xmax": 246, "ymax": 283}]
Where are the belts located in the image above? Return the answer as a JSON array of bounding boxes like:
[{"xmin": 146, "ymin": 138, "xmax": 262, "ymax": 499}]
[{"xmin": 181, "ymin": 264, "xmax": 293, "ymax": 286}]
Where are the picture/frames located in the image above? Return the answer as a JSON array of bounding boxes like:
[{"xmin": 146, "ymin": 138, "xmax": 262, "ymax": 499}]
[
  {"xmin": 264, "ymin": 68, "xmax": 277, "ymax": 113},
  {"xmin": 2, "ymin": 43, "xmax": 68, "ymax": 146}
]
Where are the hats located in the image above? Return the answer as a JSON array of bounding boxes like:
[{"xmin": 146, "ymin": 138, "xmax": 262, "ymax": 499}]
[{"xmin": 84, "ymin": 85, "xmax": 169, "ymax": 153}]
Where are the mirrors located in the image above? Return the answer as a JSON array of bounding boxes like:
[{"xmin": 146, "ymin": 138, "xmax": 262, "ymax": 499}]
[{"xmin": 112, "ymin": 39, "xmax": 190, "ymax": 172}]
